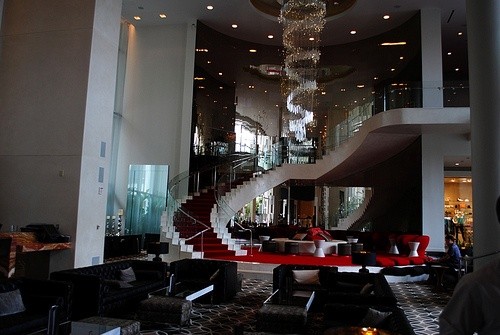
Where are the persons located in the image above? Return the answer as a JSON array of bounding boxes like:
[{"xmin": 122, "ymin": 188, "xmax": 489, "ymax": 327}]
[
  {"xmin": 426, "ymin": 235, "xmax": 463, "ymax": 284},
  {"xmin": 444, "ymin": 211, "xmax": 473, "ymax": 244},
  {"xmin": 194, "ymin": 126, "xmax": 205, "ymax": 155},
  {"xmin": 438, "ymin": 196, "xmax": 500, "ymax": 335}
]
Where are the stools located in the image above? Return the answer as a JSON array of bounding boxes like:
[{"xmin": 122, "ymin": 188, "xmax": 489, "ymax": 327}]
[
  {"xmin": 348, "ymin": 238, "xmax": 358, "ymax": 244},
  {"xmin": 258, "ymin": 236, "xmax": 270, "ymax": 248},
  {"xmin": 313, "ymin": 239, "xmax": 325, "ymax": 257},
  {"xmin": 257, "ymin": 305, "xmax": 308, "ymax": 332},
  {"xmin": 142, "ymin": 296, "xmax": 191, "ymax": 325},
  {"xmin": 79, "ymin": 316, "xmax": 140, "ymax": 335},
  {"xmin": 408, "ymin": 242, "xmax": 419, "ymax": 256},
  {"xmin": 389, "ymin": 239, "xmax": 399, "ymax": 255}
]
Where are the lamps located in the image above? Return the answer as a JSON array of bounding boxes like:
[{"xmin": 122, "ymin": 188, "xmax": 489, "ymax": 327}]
[
  {"xmin": 106, "ymin": 216, "xmax": 110, "ymax": 236},
  {"xmin": 111, "ymin": 216, "xmax": 115, "ymax": 236},
  {"xmin": 117, "ymin": 208, "xmax": 124, "ymax": 236},
  {"xmin": 279, "ymin": 0, "xmax": 327, "ymax": 144}
]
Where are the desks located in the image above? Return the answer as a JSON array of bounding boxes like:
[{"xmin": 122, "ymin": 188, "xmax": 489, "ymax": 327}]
[
  {"xmin": 104, "ymin": 235, "xmax": 142, "ymax": 256},
  {"xmin": 0, "ymin": 233, "xmax": 72, "ymax": 279},
  {"xmin": 428, "ymin": 263, "xmax": 447, "ymax": 292}
]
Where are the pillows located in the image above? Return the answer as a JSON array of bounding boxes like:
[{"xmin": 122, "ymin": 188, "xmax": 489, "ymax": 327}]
[
  {"xmin": 362, "ymin": 308, "xmax": 392, "ymax": 329},
  {"xmin": 118, "ymin": 267, "xmax": 136, "ymax": 282},
  {"xmin": 0, "ymin": 289, "xmax": 25, "ymax": 317},
  {"xmin": 105, "ymin": 280, "xmax": 133, "ymax": 288},
  {"xmin": 293, "ymin": 269, "xmax": 320, "ymax": 285},
  {"xmin": 359, "ymin": 282, "xmax": 373, "ymax": 295}
]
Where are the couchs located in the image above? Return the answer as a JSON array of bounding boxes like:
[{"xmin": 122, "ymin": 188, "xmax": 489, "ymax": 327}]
[
  {"xmin": 273, "ymin": 264, "xmax": 337, "ymax": 303},
  {"xmin": 170, "ymin": 259, "xmax": 237, "ymax": 302},
  {"xmin": 50, "ymin": 258, "xmax": 167, "ymax": 318},
  {"xmin": 0, "ymin": 276, "xmax": 72, "ymax": 335},
  {"xmin": 320, "ymin": 271, "xmax": 414, "ymax": 335}
]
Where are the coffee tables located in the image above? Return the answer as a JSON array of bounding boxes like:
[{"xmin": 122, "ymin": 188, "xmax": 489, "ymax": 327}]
[
  {"xmin": 148, "ymin": 280, "xmax": 215, "ymax": 318},
  {"xmin": 256, "ymin": 288, "xmax": 315, "ymax": 311},
  {"xmin": 30, "ymin": 321, "xmax": 120, "ymax": 335}
]
